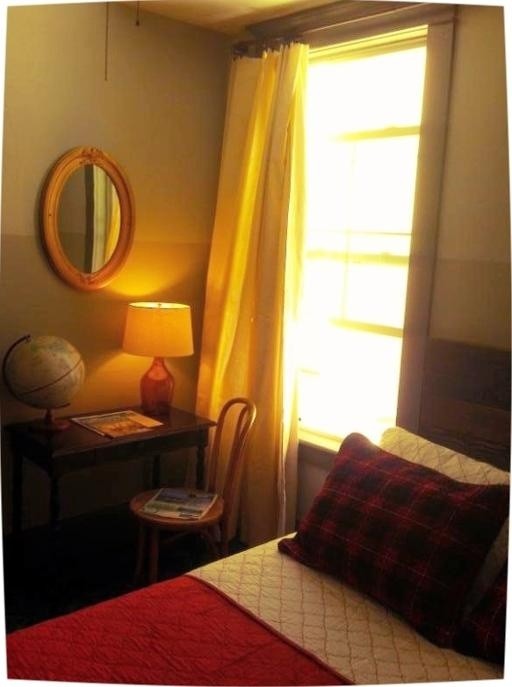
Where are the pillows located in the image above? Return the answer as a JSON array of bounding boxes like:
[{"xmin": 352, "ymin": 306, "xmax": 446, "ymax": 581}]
[
  {"xmin": 278, "ymin": 431, "xmax": 508, "ymax": 671},
  {"xmin": 452, "ymin": 559, "xmax": 507, "ymax": 666},
  {"xmin": 377, "ymin": 424, "xmax": 508, "ymax": 619}
]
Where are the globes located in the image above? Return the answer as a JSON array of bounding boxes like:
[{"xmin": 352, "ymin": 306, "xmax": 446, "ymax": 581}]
[{"xmin": 2, "ymin": 334, "xmax": 85, "ymax": 431}]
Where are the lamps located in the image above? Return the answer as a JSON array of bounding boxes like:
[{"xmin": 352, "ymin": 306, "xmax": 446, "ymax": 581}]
[{"xmin": 119, "ymin": 300, "xmax": 197, "ymax": 417}]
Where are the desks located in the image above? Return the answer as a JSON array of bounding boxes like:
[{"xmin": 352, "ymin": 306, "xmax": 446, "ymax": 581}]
[{"xmin": 7, "ymin": 400, "xmax": 217, "ymax": 573}]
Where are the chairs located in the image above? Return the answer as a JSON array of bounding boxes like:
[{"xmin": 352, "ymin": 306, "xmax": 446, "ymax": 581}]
[{"xmin": 132, "ymin": 394, "xmax": 259, "ymax": 586}]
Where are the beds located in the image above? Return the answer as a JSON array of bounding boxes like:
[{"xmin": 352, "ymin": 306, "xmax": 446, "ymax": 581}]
[{"xmin": 5, "ymin": 333, "xmax": 509, "ymax": 685}]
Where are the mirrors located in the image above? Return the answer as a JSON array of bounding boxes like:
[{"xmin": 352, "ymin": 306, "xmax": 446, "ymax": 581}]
[{"xmin": 38, "ymin": 145, "xmax": 136, "ymax": 294}]
